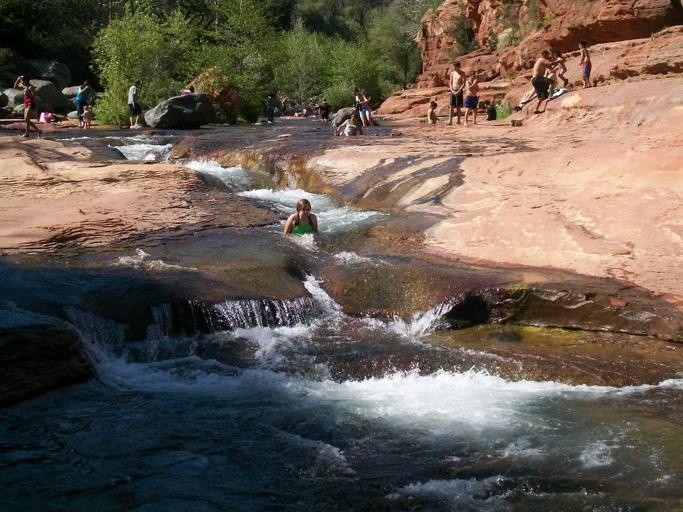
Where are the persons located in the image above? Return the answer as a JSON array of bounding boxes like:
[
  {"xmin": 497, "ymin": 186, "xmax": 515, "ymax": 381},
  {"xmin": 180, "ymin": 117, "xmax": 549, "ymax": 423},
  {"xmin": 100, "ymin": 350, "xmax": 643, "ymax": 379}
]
[
  {"xmin": 280, "ymin": 97, "xmax": 291, "ymax": 111},
  {"xmin": 578, "ymin": 40, "xmax": 593, "ymax": 89},
  {"xmin": 350, "ymin": 86, "xmax": 373, "ymax": 127},
  {"xmin": 445, "ymin": 60, "xmax": 466, "ymax": 126},
  {"xmin": 520, "ymin": 55, "xmax": 558, "ymax": 103},
  {"xmin": 362, "ymin": 90, "xmax": 372, "ymax": 119},
  {"xmin": 303, "ymin": 107, "xmax": 310, "ymax": 117},
  {"xmin": 530, "ymin": 49, "xmax": 563, "ymax": 114},
  {"xmin": 80, "ymin": 106, "xmax": 92, "ymax": 129},
  {"xmin": 427, "ymin": 100, "xmax": 441, "ymax": 124},
  {"xmin": 316, "ymin": 97, "xmax": 332, "ymax": 123},
  {"xmin": 314, "ymin": 104, "xmax": 323, "ymax": 119},
  {"xmin": 336, "ymin": 113, "xmax": 363, "ymax": 136},
  {"xmin": 551, "ymin": 49, "xmax": 570, "ymax": 86},
  {"xmin": 464, "ymin": 70, "xmax": 479, "ymax": 126},
  {"xmin": 75, "ymin": 80, "xmax": 94, "ymax": 128},
  {"xmin": 180, "ymin": 85, "xmax": 194, "ymax": 96},
  {"xmin": 39, "ymin": 107, "xmax": 65, "ymax": 123},
  {"xmin": 14, "ymin": 75, "xmax": 43, "ymax": 138},
  {"xmin": 264, "ymin": 93, "xmax": 277, "ymax": 124},
  {"xmin": 483, "ymin": 100, "xmax": 495, "ymax": 114},
  {"xmin": 282, "ymin": 198, "xmax": 320, "ymax": 237},
  {"xmin": 127, "ymin": 79, "xmax": 142, "ymax": 129}
]
